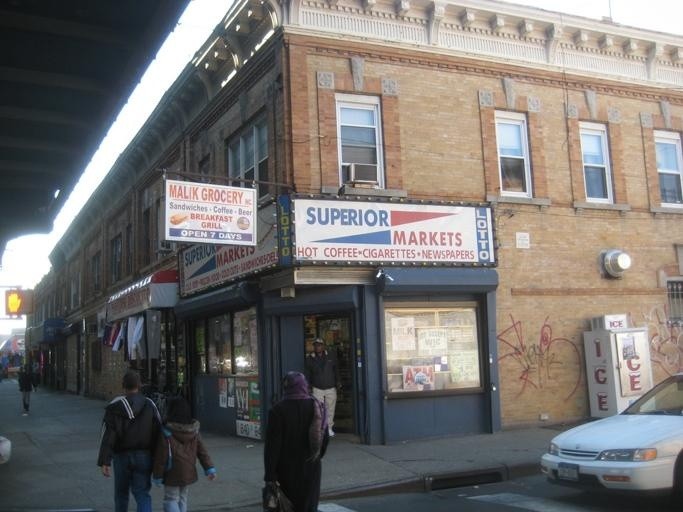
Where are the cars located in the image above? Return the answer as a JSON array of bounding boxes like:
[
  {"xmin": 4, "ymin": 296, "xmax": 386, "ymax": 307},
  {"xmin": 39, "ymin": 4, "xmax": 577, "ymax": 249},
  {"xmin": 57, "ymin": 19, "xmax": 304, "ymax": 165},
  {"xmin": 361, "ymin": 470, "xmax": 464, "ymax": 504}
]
[
  {"xmin": 540, "ymin": 371, "xmax": 682, "ymax": 512},
  {"xmin": 202, "ymin": 353, "xmax": 252, "ymax": 376}
]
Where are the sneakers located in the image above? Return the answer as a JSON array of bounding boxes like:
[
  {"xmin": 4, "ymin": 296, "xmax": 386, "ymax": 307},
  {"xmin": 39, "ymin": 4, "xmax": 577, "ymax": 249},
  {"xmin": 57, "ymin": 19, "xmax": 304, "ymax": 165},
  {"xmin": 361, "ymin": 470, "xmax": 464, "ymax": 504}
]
[{"xmin": 328, "ymin": 429, "xmax": 335, "ymax": 436}]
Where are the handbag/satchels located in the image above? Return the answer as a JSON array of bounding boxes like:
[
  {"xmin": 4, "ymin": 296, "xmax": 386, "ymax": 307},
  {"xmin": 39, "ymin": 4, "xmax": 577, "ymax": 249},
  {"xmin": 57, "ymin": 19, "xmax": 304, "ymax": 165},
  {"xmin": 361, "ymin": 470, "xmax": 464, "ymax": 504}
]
[{"xmin": 262, "ymin": 481, "xmax": 296, "ymax": 512}]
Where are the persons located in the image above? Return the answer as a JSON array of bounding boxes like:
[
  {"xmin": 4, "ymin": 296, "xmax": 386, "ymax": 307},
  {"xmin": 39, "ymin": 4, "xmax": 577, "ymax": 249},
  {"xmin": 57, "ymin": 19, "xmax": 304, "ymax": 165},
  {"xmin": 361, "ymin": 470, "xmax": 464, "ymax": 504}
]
[
  {"xmin": 18, "ymin": 361, "xmax": 38, "ymax": 413},
  {"xmin": 306, "ymin": 334, "xmax": 344, "ymax": 440},
  {"xmin": 94, "ymin": 369, "xmax": 162, "ymax": 511},
  {"xmin": 263, "ymin": 370, "xmax": 328, "ymax": 511},
  {"xmin": 152, "ymin": 394, "xmax": 217, "ymax": 511}
]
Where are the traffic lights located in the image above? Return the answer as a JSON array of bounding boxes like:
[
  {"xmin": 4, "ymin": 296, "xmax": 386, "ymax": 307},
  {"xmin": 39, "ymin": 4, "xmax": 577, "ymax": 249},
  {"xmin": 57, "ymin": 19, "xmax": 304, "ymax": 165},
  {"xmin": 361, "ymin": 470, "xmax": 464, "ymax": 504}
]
[{"xmin": 5, "ymin": 289, "xmax": 36, "ymax": 316}]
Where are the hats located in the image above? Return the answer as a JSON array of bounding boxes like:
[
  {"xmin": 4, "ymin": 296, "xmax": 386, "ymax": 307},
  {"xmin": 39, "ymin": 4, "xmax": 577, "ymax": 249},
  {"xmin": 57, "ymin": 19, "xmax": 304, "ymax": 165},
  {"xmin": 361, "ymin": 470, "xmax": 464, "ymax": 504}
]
[
  {"xmin": 283, "ymin": 371, "xmax": 307, "ymax": 393},
  {"xmin": 311, "ymin": 336, "xmax": 326, "ymax": 344}
]
